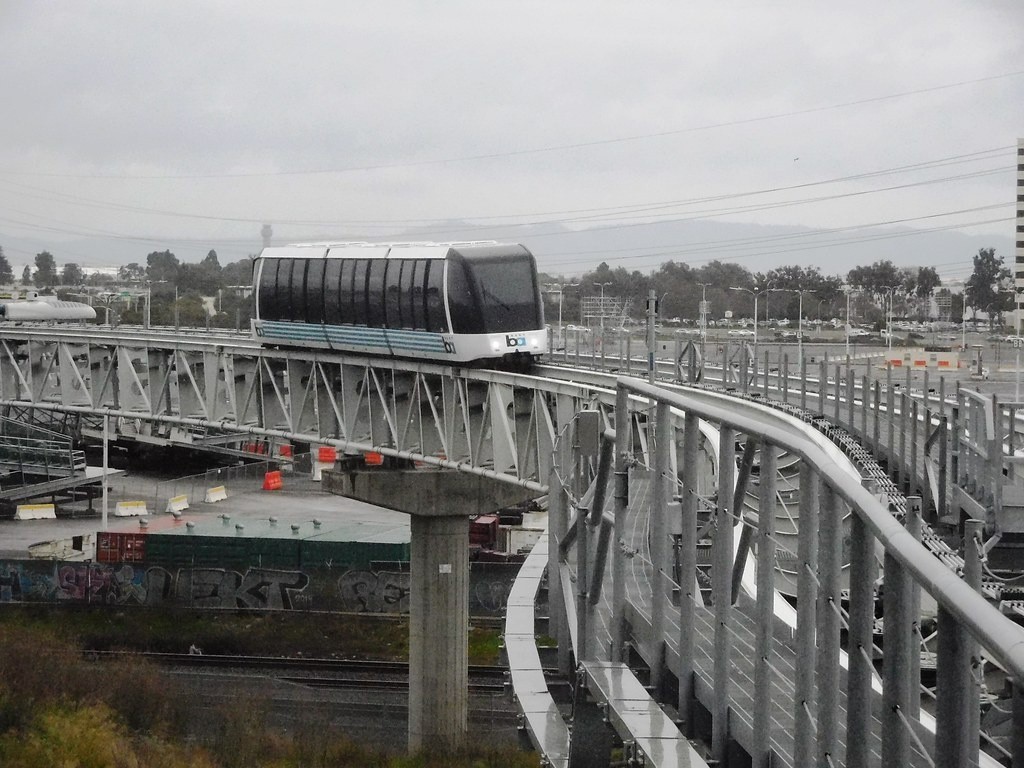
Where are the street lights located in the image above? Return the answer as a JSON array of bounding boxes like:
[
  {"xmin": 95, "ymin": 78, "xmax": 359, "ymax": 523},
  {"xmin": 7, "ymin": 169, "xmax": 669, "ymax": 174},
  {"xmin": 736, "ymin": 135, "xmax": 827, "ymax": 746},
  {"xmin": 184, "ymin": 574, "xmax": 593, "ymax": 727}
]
[
  {"xmin": 878, "ymin": 284, "xmax": 899, "ymax": 352},
  {"xmin": 730, "ymin": 285, "xmax": 774, "ymax": 344},
  {"xmin": 835, "ymin": 288, "xmax": 860, "ymax": 356},
  {"xmin": 593, "ymin": 281, "xmax": 614, "ymax": 352},
  {"xmin": 542, "ymin": 281, "xmax": 582, "ymax": 346},
  {"xmin": 694, "ymin": 283, "xmax": 712, "ymax": 338},
  {"xmin": 785, "ymin": 288, "xmax": 817, "ymax": 334},
  {"xmin": 131, "ymin": 280, "xmax": 169, "ymax": 330}
]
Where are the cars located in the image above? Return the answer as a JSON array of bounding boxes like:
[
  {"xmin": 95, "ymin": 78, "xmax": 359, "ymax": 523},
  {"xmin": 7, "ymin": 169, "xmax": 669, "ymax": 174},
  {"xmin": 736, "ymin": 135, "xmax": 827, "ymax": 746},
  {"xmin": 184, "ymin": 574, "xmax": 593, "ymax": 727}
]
[{"xmin": 546, "ymin": 317, "xmax": 1024, "ymax": 344}]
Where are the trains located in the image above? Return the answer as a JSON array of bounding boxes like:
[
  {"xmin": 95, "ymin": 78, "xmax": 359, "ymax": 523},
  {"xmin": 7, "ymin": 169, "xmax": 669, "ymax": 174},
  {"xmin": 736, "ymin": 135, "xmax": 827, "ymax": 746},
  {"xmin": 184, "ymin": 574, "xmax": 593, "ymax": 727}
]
[{"xmin": 250, "ymin": 237, "xmax": 548, "ymax": 374}]
